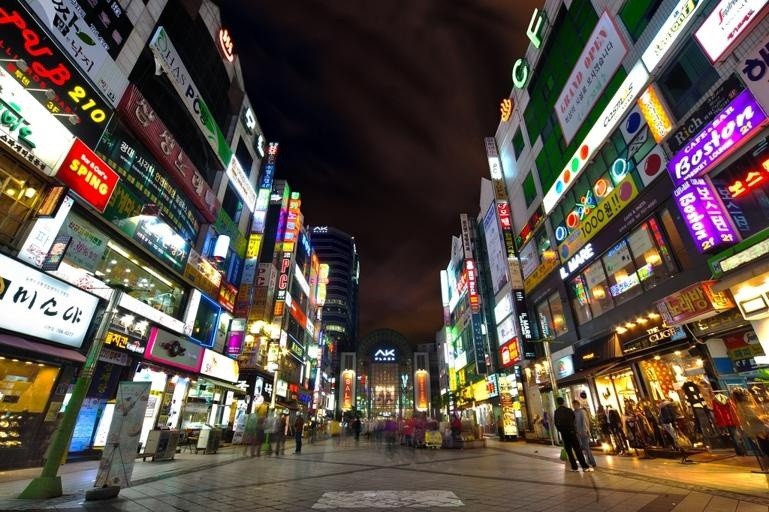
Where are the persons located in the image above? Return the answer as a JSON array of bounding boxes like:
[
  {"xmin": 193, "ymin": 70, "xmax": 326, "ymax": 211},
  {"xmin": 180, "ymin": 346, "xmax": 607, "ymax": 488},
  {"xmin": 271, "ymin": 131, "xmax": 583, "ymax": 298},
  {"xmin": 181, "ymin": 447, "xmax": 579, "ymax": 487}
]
[
  {"xmin": 554, "ymin": 397, "xmax": 596, "ymax": 473},
  {"xmin": 243, "ymin": 411, "xmax": 304, "ymax": 458},
  {"xmin": 350, "ymin": 416, "xmax": 427, "ymax": 451}
]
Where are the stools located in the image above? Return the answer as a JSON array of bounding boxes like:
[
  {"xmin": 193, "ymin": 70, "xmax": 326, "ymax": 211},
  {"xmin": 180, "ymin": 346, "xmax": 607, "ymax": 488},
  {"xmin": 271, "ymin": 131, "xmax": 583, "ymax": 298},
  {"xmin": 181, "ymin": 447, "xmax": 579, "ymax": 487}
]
[{"xmin": 183, "ymin": 436, "xmax": 199, "ymax": 454}]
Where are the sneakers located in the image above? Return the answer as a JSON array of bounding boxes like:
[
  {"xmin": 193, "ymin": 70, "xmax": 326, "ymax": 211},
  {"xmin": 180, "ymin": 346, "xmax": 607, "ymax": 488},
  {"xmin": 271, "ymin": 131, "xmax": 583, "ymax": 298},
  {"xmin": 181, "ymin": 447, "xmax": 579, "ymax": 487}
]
[
  {"xmin": 571, "ymin": 469, "xmax": 579, "ymax": 471},
  {"xmin": 584, "ymin": 467, "xmax": 594, "ymax": 471}
]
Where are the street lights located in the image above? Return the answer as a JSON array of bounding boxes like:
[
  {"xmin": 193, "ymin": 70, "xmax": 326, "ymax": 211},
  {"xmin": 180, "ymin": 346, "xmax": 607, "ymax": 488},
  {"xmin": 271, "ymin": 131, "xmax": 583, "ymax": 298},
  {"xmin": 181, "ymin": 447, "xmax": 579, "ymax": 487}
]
[
  {"xmin": 15, "ymin": 282, "xmax": 136, "ymax": 501},
  {"xmin": 524, "ymin": 337, "xmax": 577, "ymax": 461},
  {"xmin": 259, "ymin": 368, "xmax": 283, "ymax": 457}
]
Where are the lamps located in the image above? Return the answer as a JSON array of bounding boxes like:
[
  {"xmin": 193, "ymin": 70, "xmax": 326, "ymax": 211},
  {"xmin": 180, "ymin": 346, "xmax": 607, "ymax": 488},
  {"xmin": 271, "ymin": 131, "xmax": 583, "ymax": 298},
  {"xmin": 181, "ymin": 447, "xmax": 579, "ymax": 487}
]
[{"xmin": 212, "ymin": 234, "xmax": 230, "ymax": 263}]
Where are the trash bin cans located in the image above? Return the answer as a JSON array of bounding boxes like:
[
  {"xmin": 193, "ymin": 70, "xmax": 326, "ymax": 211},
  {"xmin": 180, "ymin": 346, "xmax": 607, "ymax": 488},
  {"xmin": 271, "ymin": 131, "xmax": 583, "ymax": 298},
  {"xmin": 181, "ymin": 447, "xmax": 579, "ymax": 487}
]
[{"xmin": 533, "ymin": 414, "xmax": 546, "ymax": 441}]
